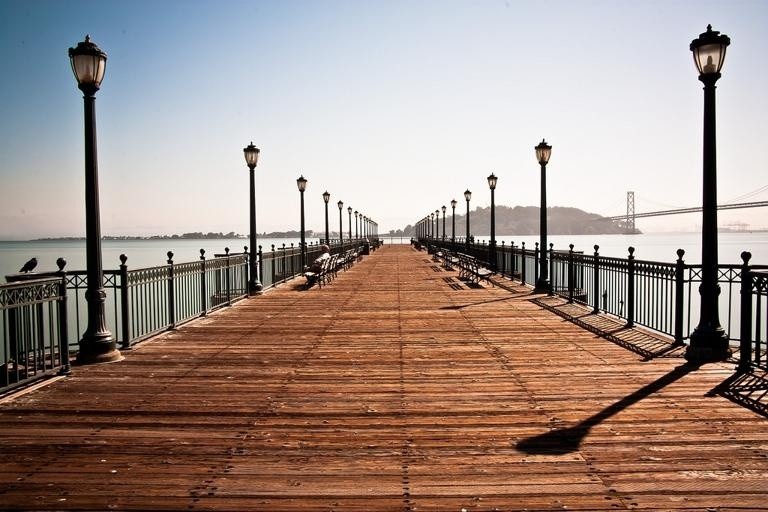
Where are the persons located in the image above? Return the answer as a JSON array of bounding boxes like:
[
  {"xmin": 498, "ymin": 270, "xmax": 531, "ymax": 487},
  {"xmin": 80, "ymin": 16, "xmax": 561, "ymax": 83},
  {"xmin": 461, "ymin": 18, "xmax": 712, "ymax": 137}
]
[{"xmin": 303, "ymin": 244, "xmax": 331, "ymax": 286}]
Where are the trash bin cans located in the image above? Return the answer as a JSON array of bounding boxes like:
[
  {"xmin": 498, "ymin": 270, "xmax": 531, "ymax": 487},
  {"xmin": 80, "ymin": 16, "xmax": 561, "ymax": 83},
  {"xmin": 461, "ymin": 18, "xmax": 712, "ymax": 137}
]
[{"xmin": 362, "ymin": 243, "xmax": 369, "ymax": 255}]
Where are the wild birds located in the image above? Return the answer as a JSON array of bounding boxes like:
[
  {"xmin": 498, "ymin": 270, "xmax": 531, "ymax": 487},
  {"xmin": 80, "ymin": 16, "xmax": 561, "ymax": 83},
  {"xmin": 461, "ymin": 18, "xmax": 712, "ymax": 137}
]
[{"xmin": 19, "ymin": 258, "xmax": 37, "ymax": 272}]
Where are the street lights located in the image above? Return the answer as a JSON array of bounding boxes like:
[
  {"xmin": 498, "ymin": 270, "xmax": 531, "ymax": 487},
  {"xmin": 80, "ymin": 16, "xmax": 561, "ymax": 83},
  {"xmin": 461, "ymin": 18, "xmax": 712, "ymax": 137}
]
[
  {"xmin": 243, "ymin": 141, "xmax": 265, "ymax": 291},
  {"xmin": 451, "ymin": 200, "xmax": 457, "ymax": 252},
  {"xmin": 431, "ymin": 213, "xmax": 434, "ymax": 240},
  {"xmin": 347, "ymin": 207, "xmax": 352, "ymax": 242},
  {"xmin": 337, "ymin": 200, "xmax": 343, "ymax": 244},
  {"xmin": 354, "ymin": 211, "xmax": 358, "ymax": 238},
  {"xmin": 488, "ymin": 173, "xmax": 499, "ymax": 270},
  {"xmin": 322, "ymin": 190, "xmax": 329, "ymax": 246},
  {"xmin": 689, "ymin": 25, "xmax": 731, "ymax": 349},
  {"xmin": 436, "ymin": 210, "xmax": 439, "ymax": 240},
  {"xmin": 296, "ymin": 175, "xmax": 308, "ymax": 274},
  {"xmin": 416, "ymin": 216, "xmax": 430, "ymax": 239},
  {"xmin": 464, "ymin": 189, "xmax": 472, "ymax": 254},
  {"xmin": 533, "ymin": 139, "xmax": 551, "ymax": 288},
  {"xmin": 442, "ymin": 206, "xmax": 446, "ymax": 242},
  {"xmin": 359, "ymin": 214, "xmax": 378, "ymax": 239},
  {"xmin": 68, "ymin": 37, "xmax": 115, "ymax": 357}
]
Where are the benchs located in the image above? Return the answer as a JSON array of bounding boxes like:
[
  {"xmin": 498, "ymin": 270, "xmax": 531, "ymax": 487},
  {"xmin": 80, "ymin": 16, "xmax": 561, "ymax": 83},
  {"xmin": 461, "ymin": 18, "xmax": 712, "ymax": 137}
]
[
  {"xmin": 411, "ymin": 239, "xmax": 495, "ymax": 286},
  {"xmin": 304, "ymin": 239, "xmax": 384, "ymax": 289}
]
